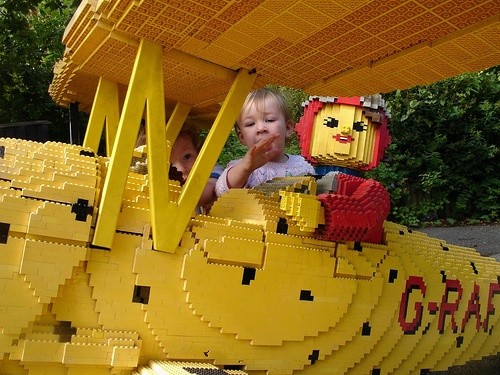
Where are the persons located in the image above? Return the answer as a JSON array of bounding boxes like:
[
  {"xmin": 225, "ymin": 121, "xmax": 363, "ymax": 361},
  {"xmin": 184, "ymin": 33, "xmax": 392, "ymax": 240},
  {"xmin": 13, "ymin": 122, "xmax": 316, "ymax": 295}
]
[
  {"xmin": 214, "ymin": 88, "xmax": 316, "ymax": 198},
  {"xmin": 133, "ymin": 121, "xmax": 219, "ymax": 216}
]
